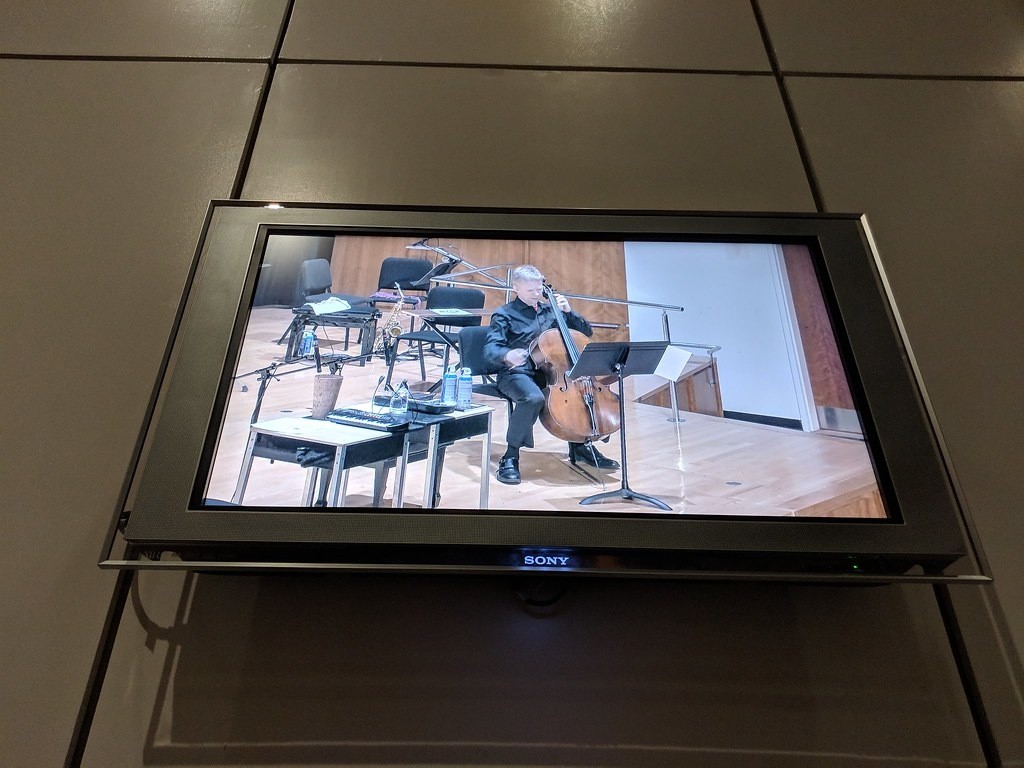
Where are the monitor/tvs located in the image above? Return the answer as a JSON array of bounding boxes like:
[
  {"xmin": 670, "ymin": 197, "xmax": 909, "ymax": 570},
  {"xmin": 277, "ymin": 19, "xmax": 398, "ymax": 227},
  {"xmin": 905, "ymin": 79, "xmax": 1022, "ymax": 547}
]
[{"xmin": 97, "ymin": 198, "xmax": 994, "ymax": 584}]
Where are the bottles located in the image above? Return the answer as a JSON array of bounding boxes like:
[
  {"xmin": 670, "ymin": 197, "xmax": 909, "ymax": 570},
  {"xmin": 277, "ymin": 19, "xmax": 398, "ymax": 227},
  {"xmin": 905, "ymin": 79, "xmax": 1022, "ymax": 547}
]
[
  {"xmin": 306, "ymin": 333, "xmax": 313, "ymax": 354},
  {"xmin": 298, "ymin": 332, "xmax": 307, "ymax": 356},
  {"xmin": 441, "ymin": 365, "xmax": 456, "ymax": 402},
  {"xmin": 456, "ymin": 367, "xmax": 472, "ymax": 410}
]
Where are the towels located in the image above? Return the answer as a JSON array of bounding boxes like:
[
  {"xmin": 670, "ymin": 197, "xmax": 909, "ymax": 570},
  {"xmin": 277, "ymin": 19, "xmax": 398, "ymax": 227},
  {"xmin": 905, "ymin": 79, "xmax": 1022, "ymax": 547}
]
[{"xmin": 303, "ymin": 296, "xmax": 352, "ymax": 316}]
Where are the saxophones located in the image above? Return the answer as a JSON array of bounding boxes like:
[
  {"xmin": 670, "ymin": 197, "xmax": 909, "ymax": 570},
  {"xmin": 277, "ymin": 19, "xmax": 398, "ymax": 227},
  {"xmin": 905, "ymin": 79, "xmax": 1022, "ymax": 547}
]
[{"xmin": 375, "ymin": 280, "xmax": 405, "ymax": 354}]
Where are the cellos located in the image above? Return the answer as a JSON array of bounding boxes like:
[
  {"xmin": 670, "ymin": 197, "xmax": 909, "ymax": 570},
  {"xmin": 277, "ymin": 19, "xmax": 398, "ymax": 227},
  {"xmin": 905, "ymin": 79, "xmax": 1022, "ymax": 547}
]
[{"xmin": 528, "ymin": 280, "xmax": 625, "ymax": 493}]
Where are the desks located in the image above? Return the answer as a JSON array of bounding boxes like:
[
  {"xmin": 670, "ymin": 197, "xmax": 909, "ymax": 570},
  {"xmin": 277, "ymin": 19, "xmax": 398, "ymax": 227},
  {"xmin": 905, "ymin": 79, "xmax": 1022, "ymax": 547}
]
[
  {"xmin": 403, "ymin": 307, "xmax": 498, "ymax": 393},
  {"xmin": 285, "ymin": 304, "xmax": 378, "ymax": 367},
  {"xmin": 233, "ymin": 402, "xmax": 496, "ymax": 509}
]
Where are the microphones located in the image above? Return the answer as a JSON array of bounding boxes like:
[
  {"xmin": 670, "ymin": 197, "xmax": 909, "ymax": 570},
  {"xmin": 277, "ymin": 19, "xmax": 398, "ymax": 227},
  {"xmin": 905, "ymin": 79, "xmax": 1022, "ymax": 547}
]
[
  {"xmin": 413, "ymin": 239, "xmax": 429, "ymax": 246},
  {"xmin": 313, "ymin": 334, "xmax": 322, "ymax": 372},
  {"xmin": 382, "ymin": 328, "xmax": 391, "ymax": 367}
]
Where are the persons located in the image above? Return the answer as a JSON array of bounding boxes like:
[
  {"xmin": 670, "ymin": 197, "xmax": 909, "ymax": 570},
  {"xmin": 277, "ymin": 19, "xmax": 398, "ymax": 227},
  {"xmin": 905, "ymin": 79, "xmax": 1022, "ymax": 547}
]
[{"xmin": 482, "ymin": 264, "xmax": 619, "ymax": 484}]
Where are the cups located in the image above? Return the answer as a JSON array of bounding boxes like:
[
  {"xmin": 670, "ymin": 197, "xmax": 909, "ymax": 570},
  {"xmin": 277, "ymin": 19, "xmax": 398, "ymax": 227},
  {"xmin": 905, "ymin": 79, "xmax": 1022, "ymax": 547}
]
[
  {"xmin": 389, "ymin": 384, "xmax": 408, "ymax": 413},
  {"xmin": 312, "ymin": 374, "xmax": 343, "ymax": 418}
]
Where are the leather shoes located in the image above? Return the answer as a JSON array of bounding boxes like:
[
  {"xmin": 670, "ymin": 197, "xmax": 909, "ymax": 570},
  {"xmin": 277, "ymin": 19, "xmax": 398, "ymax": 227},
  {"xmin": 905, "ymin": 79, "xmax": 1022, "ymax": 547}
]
[
  {"xmin": 496, "ymin": 456, "xmax": 521, "ymax": 483},
  {"xmin": 569, "ymin": 444, "xmax": 620, "ymax": 469}
]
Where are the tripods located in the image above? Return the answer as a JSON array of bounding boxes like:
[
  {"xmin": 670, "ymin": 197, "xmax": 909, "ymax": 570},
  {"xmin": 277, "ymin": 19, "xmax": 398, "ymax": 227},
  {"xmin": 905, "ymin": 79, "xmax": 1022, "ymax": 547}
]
[
  {"xmin": 565, "ymin": 341, "xmax": 673, "ymax": 511},
  {"xmin": 399, "ymin": 263, "xmax": 457, "ymax": 361}
]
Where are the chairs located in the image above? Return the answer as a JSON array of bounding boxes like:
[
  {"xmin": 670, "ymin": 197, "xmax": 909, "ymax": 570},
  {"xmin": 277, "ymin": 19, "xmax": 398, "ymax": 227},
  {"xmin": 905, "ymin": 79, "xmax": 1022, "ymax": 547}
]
[
  {"xmin": 458, "ymin": 325, "xmax": 575, "ymax": 464},
  {"xmin": 278, "ymin": 259, "xmax": 366, "ymax": 351},
  {"xmin": 384, "ymin": 286, "xmax": 487, "ymax": 391},
  {"xmin": 358, "ymin": 258, "xmax": 433, "ymax": 346}
]
